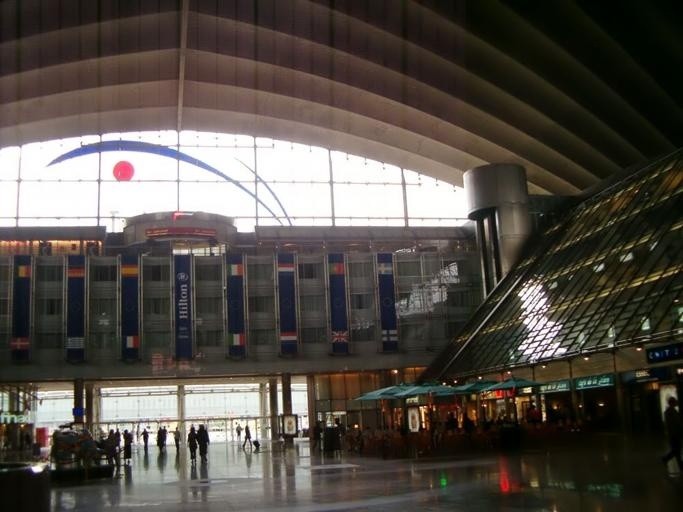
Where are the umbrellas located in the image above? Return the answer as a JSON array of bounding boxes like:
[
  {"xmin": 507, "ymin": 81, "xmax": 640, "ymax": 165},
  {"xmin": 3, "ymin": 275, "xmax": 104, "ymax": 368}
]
[{"xmin": 352, "ymin": 374, "xmax": 547, "ymax": 422}]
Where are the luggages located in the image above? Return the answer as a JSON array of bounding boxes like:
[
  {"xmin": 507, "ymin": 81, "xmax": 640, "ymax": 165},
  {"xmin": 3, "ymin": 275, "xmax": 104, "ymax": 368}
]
[{"xmin": 252, "ymin": 440, "xmax": 260, "ymax": 448}]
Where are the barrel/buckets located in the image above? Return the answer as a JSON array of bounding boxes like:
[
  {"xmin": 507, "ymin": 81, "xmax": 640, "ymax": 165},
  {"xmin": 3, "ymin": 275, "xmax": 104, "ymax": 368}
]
[{"xmin": 36, "ymin": 426, "xmax": 49, "ymax": 448}]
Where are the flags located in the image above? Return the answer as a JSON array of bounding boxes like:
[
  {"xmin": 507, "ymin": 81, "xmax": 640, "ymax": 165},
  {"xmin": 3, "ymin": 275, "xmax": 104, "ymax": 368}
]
[
  {"xmin": 276, "ymin": 261, "xmax": 294, "ymax": 276},
  {"xmin": 119, "ymin": 264, "xmax": 138, "ymax": 277},
  {"xmin": 120, "ymin": 334, "xmax": 140, "ymax": 350},
  {"xmin": 66, "ymin": 263, "xmax": 85, "ymax": 279},
  {"xmin": 375, "ymin": 262, "xmax": 392, "ymax": 276},
  {"xmin": 64, "ymin": 336, "xmax": 86, "ymax": 349},
  {"xmin": 228, "ymin": 331, "xmax": 246, "ymax": 348},
  {"xmin": 11, "ymin": 336, "xmax": 29, "ymax": 351},
  {"xmin": 327, "ymin": 261, "xmax": 344, "ymax": 275},
  {"xmin": 278, "ymin": 330, "xmax": 297, "ymax": 346},
  {"xmin": 226, "ymin": 262, "xmax": 243, "ymax": 276},
  {"xmin": 380, "ymin": 328, "xmax": 398, "ymax": 342},
  {"xmin": 330, "ymin": 328, "xmax": 348, "ymax": 344},
  {"xmin": 11, "ymin": 263, "xmax": 31, "ymax": 279}
]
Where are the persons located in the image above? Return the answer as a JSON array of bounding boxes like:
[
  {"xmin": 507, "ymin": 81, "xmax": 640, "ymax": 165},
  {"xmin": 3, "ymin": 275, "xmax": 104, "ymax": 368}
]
[
  {"xmin": 312, "ymin": 394, "xmax": 681, "ymax": 471},
  {"xmin": 235, "ymin": 423, "xmax": 244, "ymax": 440},
  {"xmin": 0, "ymin": 423, "xmax": 211, "ymax": 466},
  {"xmin": 241, "ymin": 425, "xmax": 252, "ymax": 447}
]
